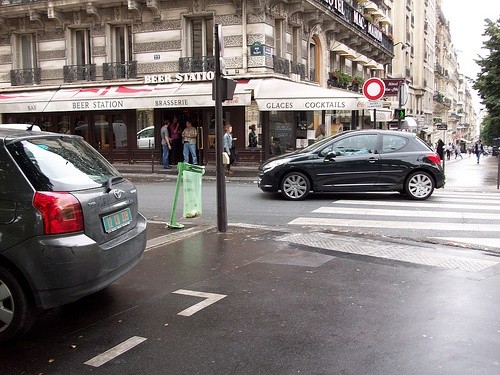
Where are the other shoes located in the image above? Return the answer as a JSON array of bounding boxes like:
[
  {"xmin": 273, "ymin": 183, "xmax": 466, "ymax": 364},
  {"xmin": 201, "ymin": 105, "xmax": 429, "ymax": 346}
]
[{"xmin": 226, "ymin": 169, "xmax": 233, "ymax": 174}]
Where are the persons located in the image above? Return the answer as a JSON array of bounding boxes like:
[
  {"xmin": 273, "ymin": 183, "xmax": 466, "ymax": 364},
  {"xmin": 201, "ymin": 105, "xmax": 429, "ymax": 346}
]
[
  {"xmin": 161, "ymin": 120, "xmax": 172, "ymax": 169},
  {"xmin": 452, "ymin": 143, "xmax": 463, "ymax": 159},
  {"xmin": 475, "ymin": 140, "xmax": 484, "ymax": 163},
  {"xmin": 248, "ymin": 124, "xmax": 258, "ymax": 147},
  {"xmin": 182, "ymin": 119, "xmax": 198, "ymax": 165},
  {"xmin": 169, "ymin": 115, "xmax": 182, "ymax": 165},
  {"xmin": 272, "ymin": 136, "xmax": 286, "ymax": 156},
  {"xmin": 437, "ymin": 139, "xmax": 444, "ymax": 160},
  {"xmin": 223, "ymin": 124, "xmax": 237, "ymax": 174},
  {"xmin": 468, "ymin": 144, "xmax": 475, "ymax": 157},
  {"xmin": 446, "ymin": 142, "xmax": 452, "ymax": 160}
]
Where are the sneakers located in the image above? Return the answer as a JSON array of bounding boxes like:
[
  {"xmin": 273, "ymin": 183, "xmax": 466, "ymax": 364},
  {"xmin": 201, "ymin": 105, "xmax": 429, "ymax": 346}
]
[{"xmin": 164, "ymin": 166, "xmax": 172, "ymax": 168}]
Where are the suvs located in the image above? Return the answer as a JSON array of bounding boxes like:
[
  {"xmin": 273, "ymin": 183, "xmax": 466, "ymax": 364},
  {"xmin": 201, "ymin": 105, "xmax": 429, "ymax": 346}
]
[
  {"xmin": 0, "ymin": 128, "xmax": 148, "ymax": 340},
  {"xmin": 256, "ymin": 129, "xmax": 447, "ymax": 201}
]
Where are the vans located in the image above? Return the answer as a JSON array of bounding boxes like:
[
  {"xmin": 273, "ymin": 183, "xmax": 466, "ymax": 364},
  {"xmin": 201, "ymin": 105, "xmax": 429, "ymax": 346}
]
[{"xmin": 75, "ymin": 123, "xmax": 127, "ymax": 148}]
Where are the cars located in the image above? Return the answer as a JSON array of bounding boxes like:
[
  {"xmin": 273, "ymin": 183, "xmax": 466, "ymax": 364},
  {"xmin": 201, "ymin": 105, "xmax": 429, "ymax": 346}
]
[{"xmin": 135, "ymin": 126, "xmax": 154, "ymax": 148}]
